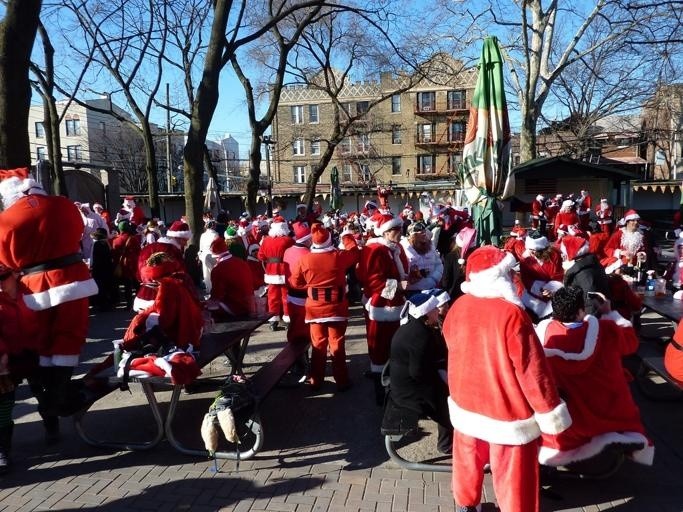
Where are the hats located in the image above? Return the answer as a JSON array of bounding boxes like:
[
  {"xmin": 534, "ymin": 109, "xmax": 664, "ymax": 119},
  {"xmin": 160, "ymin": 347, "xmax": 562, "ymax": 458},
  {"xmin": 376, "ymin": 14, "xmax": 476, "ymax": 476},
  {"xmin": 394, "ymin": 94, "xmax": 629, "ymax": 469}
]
[
  {"xmin": 1, "ymin": 165, "xmax": 43, "ymax": 198},
  {"xmin": 461, "ymin": 244, "xmax": 518, "ymax": 292},
  {"xmin": 623, "ymin": 210, "xmax": 640, "ymax": 221},
  {"xmin": 560, "ymin": 236, "xmax": 590, "ymax": 260},
  {"xmin": 118, "ymin": 221, "xmax": 131, "ymax": 232},
  {"xmin": 399, "ymin": 288, "xmax": 451, "ymax": 321},
  {"xmin": 268, "ymin": 216, "xmax": 332, "ymax": 248},
  {"xmin": 210, "ymin": 237, "xmax": 228, "ymax": 258},
  {"xmin": 166, "ymin": 220, "xmax": 190, "ymax": 238},
  {"xmin": 89, "ymin": 228, "xmax": 107, "ymax": 237},
  {"xmin": 224, "ymin": 225, "xmax": 238, "ymax": 239},
  {"xmin": 600, "ymin": 196, "xmax": 607, "ymax": 201},
  {"xmin": 510, "ymin": 220, "xmax": 526, "ymax": 240},
  {"xmin": 456, "ymin": 227, "xmax": 476, "ymax": 264},
  {"xmin": 600, "ymin": 257, "xmax": 623, "ymax": 274},
  {"xmin": 365, "ymin": 214, "xmax": 402, "ymax": 236},
  {"xmin": 524, "ymin": 231, "xmax": 549, "ymax": 250},
  {"xmin": 124, "ymin": 197, "xmax": 134, "ymax": 205}
]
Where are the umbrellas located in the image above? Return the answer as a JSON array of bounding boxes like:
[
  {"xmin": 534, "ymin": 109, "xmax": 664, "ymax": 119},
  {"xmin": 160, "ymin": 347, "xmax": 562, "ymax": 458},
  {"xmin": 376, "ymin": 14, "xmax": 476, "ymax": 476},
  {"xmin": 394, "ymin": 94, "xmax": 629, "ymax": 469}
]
[{"xmin": 457, "ymin": 35, "xmax": 516, "ymax": 246}]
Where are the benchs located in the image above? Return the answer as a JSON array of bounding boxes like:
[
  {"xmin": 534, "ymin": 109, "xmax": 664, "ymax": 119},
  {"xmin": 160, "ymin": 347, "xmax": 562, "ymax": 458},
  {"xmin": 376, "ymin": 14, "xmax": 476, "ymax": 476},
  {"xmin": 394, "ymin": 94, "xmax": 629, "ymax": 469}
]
[
  {"xmin": 379, "ymin": 404, "xmax": 419, "ymax": 470},
  {"xmin": 36, "ymin": 373, "xmax": 118, "ymax": 445},
  {"xmin": 637, "ymin": 340, "xmax": 683, "ymax": 399},
  {"xmin": 210, "ymin": 341, "xmax": 309, "ymax": 459}
]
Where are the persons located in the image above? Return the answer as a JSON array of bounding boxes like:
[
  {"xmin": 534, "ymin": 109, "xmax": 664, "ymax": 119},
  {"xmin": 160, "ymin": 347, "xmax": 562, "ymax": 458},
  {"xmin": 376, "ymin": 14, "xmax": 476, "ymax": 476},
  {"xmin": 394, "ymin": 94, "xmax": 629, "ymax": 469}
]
[
  {"xmin": 504, "ymin": 191, "xmax": 657, "ymax": 466},
  {"xmin": 665, "ymin": 210, "xmax": 682, "ymax": 381},
  {"xmin": 442, "ymin": 245, "xmax": 571, "ymax": 512},
  {"xmin": 74, "ymin": 196, "xmax": 467, "ymax": 454},
  {"xmin": 1, "ymin": 166, "xmax": 99, "ymax": 469}
]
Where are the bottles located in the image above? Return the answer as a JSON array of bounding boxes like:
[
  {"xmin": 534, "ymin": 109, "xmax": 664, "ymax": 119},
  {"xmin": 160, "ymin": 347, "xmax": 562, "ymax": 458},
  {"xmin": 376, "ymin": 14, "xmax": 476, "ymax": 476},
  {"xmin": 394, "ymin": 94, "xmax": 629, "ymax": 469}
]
[
  {"xmin": 636, "ymin": 257, "xmax": 645, "ymax": 282},
  {"xmin": 113, "ymin": 344, "xmax": 121, "ymax": 372}
]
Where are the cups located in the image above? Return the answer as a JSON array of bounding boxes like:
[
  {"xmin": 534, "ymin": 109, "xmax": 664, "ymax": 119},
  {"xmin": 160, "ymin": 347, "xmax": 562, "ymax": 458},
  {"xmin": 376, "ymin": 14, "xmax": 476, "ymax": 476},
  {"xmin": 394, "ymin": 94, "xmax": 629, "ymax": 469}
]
[{"xmin": 654, "ymin": 278, "xmax": 666, "ymax": 298}]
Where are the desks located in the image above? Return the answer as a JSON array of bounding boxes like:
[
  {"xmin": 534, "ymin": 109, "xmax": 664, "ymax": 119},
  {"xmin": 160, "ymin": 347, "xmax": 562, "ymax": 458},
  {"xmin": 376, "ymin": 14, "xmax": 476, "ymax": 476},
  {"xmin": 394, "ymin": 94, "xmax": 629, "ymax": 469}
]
[
  {"xmin": 636, "ymin": 294, "xmax": 682, "ymax": 334},
  {"xmin": 74, "ymin": 312, "xmax": 275, "ymax": 460}
]
